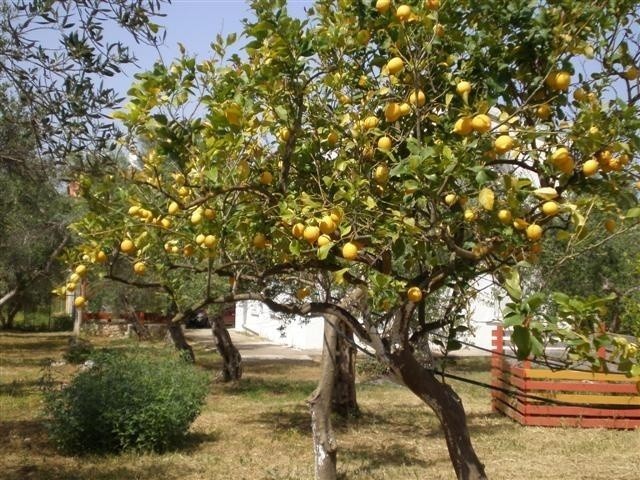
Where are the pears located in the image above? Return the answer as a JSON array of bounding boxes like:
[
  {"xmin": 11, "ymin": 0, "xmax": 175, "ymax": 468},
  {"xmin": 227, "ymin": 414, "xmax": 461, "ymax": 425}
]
[{"xmin": 67, "ymin": 1, "xmax": 640, "ymax": 307}]
